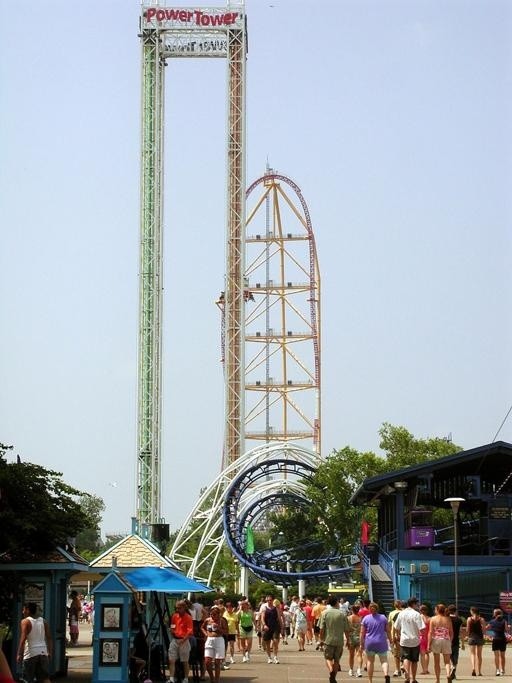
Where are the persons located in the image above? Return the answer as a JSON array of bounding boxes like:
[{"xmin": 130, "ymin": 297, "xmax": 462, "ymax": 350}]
[
  {"xmin": 167, "ymin": 595, "xmax": 238, "ymax": 683},
  {"xmin": 17, "ymin": 602, "xmax": 53, "ymax": 683},
  {"xmin": 466, "ymin": 606, "xmax": 485, "ymax": 676},
  {"xmin": 65, "ymin": 592, "xmax": 94, "ymax": 645},
  {"xmin": 486, "ymin": 609, "xmax": 509, "ymax": 676},
  {"xmin": 232, "ymin": 594, "xmax": 326, "ymax": 664},
  {"xmin": 314, "ymin": 596, "xmax": 465, "ymax": 683}
]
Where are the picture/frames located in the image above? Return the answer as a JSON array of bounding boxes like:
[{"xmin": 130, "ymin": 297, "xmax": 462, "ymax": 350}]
[
  {"xmin": 101, "ymin": 604, "xmax": 123, "ymax": 631},
  {"xmin": 22, "ymin": 581, "xmax": 45, "ymax": 601},
  {"xmin": 99, "ymin": 638, "xmax": 122, "ymax": 667},
  {"xmin": 22, "ymin": 600, "xmax": 45, "ymax": 619}
]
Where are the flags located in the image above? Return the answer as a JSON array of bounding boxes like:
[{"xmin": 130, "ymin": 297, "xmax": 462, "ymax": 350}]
[{"xmin": 245, "ymin": 525, "xmax": 254, "ymax": 554}]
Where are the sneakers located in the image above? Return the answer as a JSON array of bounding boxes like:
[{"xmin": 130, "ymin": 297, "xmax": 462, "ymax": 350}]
[
  {"xmin": 298, "ymin": 641, "xmax": 324, "ymax": 652},
  {"xmin": 267, "ymin": 656, "xmax": 273, "ymax": 663},
  {"xmin": 274, "ymin": 656, "xmax": 279, "ymax": 663},
  {"xmin": 450, "ymin": 667, "xmax": 456, "ymax": 680},
  {"xmin": 329, "ymin": 664, "xmax": 418, "ymax": 683},
  {"xmin": 472, "ymin": 669, "xmax": 476, "ymax": 676},
  {"xmin": 496, "ymin": 669, "xmax": 505, "ymax": 676},
  {"xmin": 211, "ymin": 651, "xmax": 250, "ymax": 670}
]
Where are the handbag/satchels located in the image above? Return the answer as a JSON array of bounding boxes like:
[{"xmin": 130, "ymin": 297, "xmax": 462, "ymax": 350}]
[{"xmin": 241, "ymin": 625, "xmax": 253, "ymax": 632}]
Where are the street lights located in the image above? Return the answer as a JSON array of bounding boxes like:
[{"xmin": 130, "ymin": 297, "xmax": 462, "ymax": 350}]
[{"xmin": 443, "ymin": 496, "xmax": 465, "ymax": 614}]
